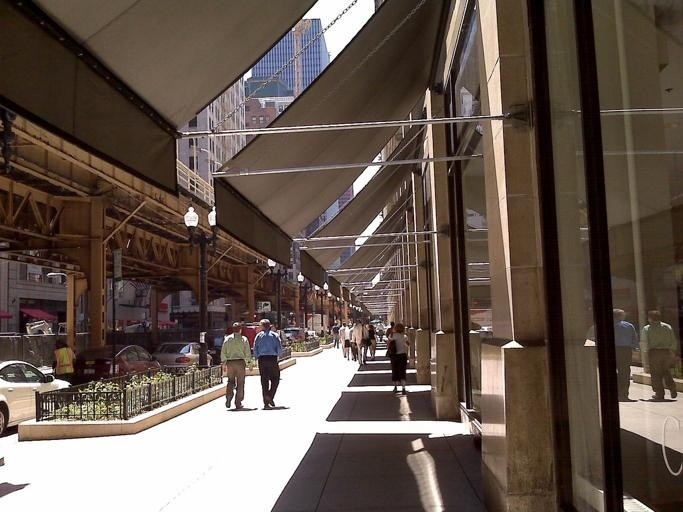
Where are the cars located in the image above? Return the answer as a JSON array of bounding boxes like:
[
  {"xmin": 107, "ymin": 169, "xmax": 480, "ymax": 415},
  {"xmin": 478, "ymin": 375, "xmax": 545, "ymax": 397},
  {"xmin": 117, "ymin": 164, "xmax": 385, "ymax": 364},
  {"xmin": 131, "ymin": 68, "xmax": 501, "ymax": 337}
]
[
  {"xmin": 149, "ymin": 341, "xmax": 214, "ymax": 376},
  {"xmin": 1, "ymin": 357, "xmax": 75, "ymax": 438},
  {"xmin": 71, "ymin": 344, "xmax": 160, "ymax": 387}
]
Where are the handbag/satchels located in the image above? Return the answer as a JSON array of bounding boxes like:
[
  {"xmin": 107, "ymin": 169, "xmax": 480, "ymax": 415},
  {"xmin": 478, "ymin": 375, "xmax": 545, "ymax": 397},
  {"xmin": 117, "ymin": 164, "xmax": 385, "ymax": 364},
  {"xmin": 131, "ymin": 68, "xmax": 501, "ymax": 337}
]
[
  {"xmin": 360, "ymin": 339, "xmax": 372, "ymax": 345},
  {"xmin": 386, "ymin": 340, "xmax": 396, "ymax": 358}
]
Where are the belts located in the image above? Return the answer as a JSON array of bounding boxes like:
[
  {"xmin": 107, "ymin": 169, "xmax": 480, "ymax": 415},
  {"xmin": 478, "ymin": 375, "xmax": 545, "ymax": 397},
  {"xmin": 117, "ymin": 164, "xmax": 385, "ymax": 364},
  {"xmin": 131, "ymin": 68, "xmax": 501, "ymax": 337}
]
[{"xmin": 227, "ymin": 359, "xmax": 244, "ymax": 361}]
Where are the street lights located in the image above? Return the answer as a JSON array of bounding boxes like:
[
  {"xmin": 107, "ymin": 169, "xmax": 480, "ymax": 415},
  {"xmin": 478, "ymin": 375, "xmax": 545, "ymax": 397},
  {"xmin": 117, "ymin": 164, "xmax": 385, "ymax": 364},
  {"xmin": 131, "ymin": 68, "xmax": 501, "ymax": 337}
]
[
  {"xmin": 267, "ymin": 258, "xmax": 284, "ymax": 331},
  {"xmin": 183, "ymin": 204, "xmax": 220, "ymax": 373},
  {"xmin": 296, "ymin": 271, "xmax": 314, "ymax": 329},
  {"xmin": 314, "ymin": 282, "xmax": 329, "ymax": 336}
]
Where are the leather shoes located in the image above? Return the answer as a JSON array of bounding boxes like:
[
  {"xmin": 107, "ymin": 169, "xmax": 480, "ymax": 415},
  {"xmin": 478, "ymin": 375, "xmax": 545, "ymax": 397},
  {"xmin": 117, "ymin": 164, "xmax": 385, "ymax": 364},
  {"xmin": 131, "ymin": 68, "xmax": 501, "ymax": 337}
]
[
  {"xmin": 225, "ymin": 400, "xmax": 244, "ymax": 410},
  {"xmin": 393, "ymin": 386, "xmax": 406, "ymax": 395},
  {"xmin": 653, "ymin": 389, "xmax": 678, "ymax": 402},
  {"xmin": 263, "ymin": 392, "xmax": 275, "ymax": 409}
]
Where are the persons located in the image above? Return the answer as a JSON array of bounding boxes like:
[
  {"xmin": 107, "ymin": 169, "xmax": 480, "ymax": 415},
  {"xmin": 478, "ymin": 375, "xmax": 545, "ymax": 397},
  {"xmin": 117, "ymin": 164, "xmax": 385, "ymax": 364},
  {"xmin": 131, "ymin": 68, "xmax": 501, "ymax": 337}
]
[
  {"xmin": 331, "ymin": 320, "xmax": 409, "ymax": 396},
  {"xmin": 219, "ymin": 319, "xmax": 283, "ymax": 410},
  {"xmin": 53, "ymin": 338, "xmax": 77, "ymax": 379},
  {"xmin": 613, "ymin": 309, "xmax": 679, "ymax": 402}
]
